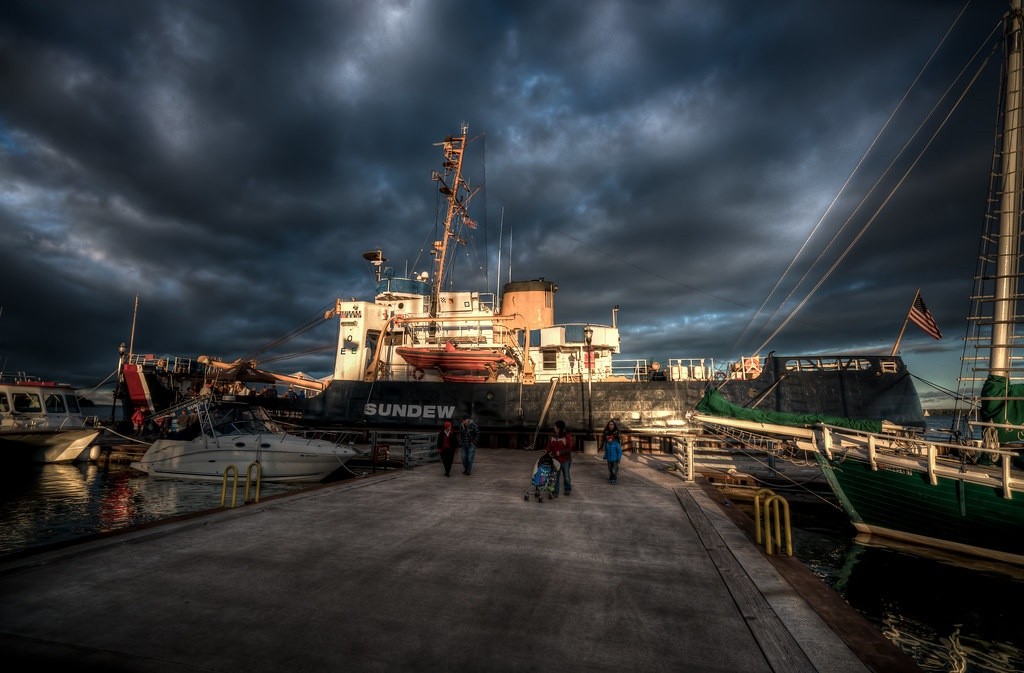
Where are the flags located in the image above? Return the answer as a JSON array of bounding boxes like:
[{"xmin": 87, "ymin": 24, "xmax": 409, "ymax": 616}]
[{"xmin": 909, "ymin": 294, "xmax": 942, "ymax": 341}]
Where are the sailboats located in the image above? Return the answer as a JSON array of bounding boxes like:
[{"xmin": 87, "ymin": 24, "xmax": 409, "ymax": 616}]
[{"xmin": 684, "ymin": 0, "xmax": 1023, "ymax": 567}]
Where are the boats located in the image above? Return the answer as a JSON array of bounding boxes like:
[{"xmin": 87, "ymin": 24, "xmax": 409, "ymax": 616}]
[
  {"xmin": 396, "ymin": 339, "xmax": 516, "ymax": 372},
  {"xmin": 130, "ymin": 367, "xmax": 365, "ymax": 482},
  {"xmin": 441, "ymin": 375, "xmax": 488, "ymax": 383},
  {"xmin": 0, "ymin": 369, "xmax": 106, "ymax": 465}
]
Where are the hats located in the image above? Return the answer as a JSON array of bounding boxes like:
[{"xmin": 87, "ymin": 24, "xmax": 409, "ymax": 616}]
[
  {"xmin": 606, "ymin": 433, "xmax": 614, "ymax": 441},
  {"xmin": 444, "ymin": 421, "xmax": 452, "ymax": 430}
]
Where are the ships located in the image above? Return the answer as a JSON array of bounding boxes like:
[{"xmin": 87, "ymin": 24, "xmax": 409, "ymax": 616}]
[{"xmin": 110, "ymin": 120, "xmax": 927, "ymax": 450}]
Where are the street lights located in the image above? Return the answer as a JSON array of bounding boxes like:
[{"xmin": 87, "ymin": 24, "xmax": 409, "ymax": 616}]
[{"xmin": 583, "ymin": 323, "xmax": 595, "ymax": 442}]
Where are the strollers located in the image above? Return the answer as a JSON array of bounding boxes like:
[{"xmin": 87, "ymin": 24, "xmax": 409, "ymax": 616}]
[{"xmin": 523, "ymin": 451, "xmax": 561, "ymax": 504}]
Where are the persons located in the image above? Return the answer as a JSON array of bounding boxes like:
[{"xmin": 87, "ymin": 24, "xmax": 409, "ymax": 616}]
[
  {"xmin": 597, "ymin": 421, "xmax": 621, "ymax": 485},
  {"xmin": 456, "ymin": 414, "xmax": 479, "ymax": 475},
  {"xmin": 532, "ymin": 462, "xmax": 551, "ymax": 486},
  {"xmin": 437, "ymin": 421, "xmax": 458, "ymax": 477},
  {"xmin": 131, "ymin": 379, "xmax": 306, "ymax": 434},
  {"xmin": 544, "ymin": 421, "xmax": 573, "ymax": 497}
]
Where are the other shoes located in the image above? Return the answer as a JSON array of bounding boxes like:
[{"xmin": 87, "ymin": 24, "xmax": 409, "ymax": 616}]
[
  {"xmin": 565, "ymin": 490, "xmax": 571, "ymax": 495},
  {"xmin": 445, "ymin": 472, "xmax": 450, "ymax": 476},
  {"xmin": 609, "ymin": 479, "xmax": 616, "ymax": 486},
  {"xmin": 552, "ymin": 493, "xmax": 558, "ymax": 498},
  {"xmin": 462, "ymin": 471, "xmax": 471, "ymax": 475}
]
